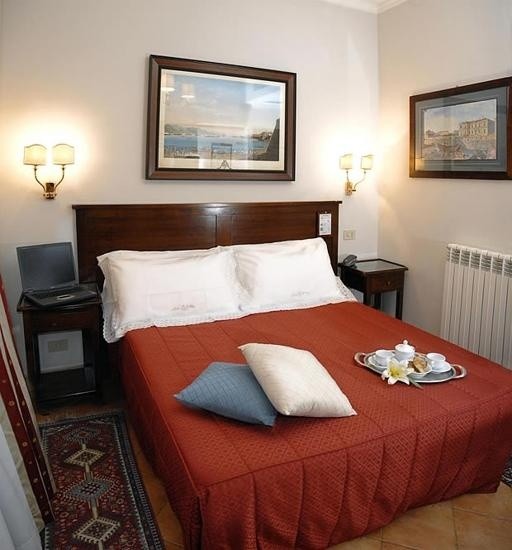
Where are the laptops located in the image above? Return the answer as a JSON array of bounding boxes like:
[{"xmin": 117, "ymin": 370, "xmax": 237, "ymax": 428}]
[{"xmin": 15, "ymin": 242, "xmax": 96, "ymax": 306}]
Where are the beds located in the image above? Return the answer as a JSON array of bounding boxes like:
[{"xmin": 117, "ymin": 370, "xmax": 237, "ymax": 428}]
[{"xmin": 71, "ymin": 200, "xmax": 512, "ymax": 549}]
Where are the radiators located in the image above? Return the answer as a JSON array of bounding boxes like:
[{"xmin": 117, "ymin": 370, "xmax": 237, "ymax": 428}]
[{"xmin": 438, "ymin": 241, "xmax": 512, "ymax": 372}]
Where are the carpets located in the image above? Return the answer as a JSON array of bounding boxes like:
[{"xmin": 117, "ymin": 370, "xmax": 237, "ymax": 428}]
[{"xmin": 35, "ymin": 408, "xmax": 169, "ymax": 549}]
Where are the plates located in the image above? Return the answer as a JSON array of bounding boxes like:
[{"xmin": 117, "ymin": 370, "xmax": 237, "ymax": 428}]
[
  {"xmin": 432, "ymin": 363, "xmax": 450, "ymax": 375},
  {"xmin": 368, "ymin": 358, "xmax": 397, "ymax": 372}
]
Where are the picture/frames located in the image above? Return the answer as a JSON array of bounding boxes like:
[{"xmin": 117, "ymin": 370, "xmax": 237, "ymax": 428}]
[
  {"xmin": 144, "ymin": 54, "xmax": 299, "ymax": 184},
  {"xmin": 407, "ymin": 75, "xmax": 512, "ymax": 184}
]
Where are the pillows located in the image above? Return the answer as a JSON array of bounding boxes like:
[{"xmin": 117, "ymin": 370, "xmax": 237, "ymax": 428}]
[
  {"xmin": 94, "ymin": 244, "xmax": 242, "ymax": 347},
  {"xmin": 237, "ymin": 339, "xmax": 359, "ymax": 421},
  {"xmin": 171, "ymin": 359, "xmax": 279, "ymax": 431},
  {"xmin": 223, "ymin": 236, "xmax": 360, "ymax": 319}
]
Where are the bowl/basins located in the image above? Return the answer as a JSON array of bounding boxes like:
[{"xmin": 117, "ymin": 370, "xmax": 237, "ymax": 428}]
[{"xmin": 401, "ymin": 360, "xmax": 431, "ymax": 377}]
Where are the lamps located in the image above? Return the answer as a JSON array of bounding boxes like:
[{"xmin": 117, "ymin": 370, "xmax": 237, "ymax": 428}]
[
  {"xmin": 338, "ymin": 150, "xmax": 376, "ymax": 197},
  {"xmin": 22, "ymin": 141, "xmax": 78, "ymax": 202}
]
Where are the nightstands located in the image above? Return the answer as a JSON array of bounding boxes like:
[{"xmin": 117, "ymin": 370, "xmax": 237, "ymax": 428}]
[
  {"xmin": 336, "ymin": 258, "xmax": 410, "ymax": 323},
  {"xmin": 15, "ymin": 282, "xmax": 104, "ymax": 413}
]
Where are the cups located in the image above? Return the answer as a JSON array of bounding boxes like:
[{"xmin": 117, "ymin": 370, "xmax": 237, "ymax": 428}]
[
  {"xmin": 392, "ymin": 339, "xmax": 415, "ymax": 362},
  {"xmin": 375, "ymin": 351, "xmax": 393, "ymax": 365},
  {"xmin": 427, "ymin": 353, "xmax": 446, "ymax": 368}
]
